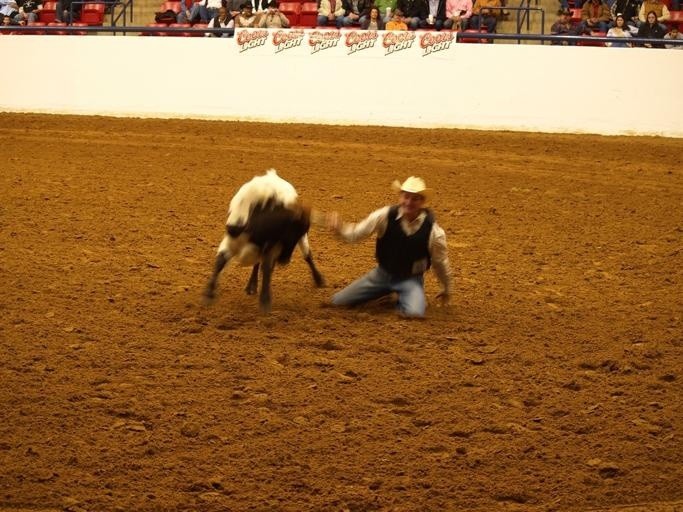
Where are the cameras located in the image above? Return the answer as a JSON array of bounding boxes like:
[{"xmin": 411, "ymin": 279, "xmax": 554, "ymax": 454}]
[{"xmin": 271, "ymin": 9, "xmax": 278, "ymax": 14}]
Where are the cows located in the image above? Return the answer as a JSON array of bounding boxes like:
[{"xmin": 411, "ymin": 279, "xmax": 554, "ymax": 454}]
[{"xmin": 200, "ymin": 167, "xmax": 324, "ymax": 317}]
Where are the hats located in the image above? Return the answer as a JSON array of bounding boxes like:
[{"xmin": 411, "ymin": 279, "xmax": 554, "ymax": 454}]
[
  {"xmin": 392, "ymin": 175, "xmax": 433, "ymax": 209},
  {"xmin": 558, "ymin": 7, "xmax": 574, "ymax": 16},
  {"xmin": 239, "ymin": 0, "xmax": 276, "ymax": 10}
]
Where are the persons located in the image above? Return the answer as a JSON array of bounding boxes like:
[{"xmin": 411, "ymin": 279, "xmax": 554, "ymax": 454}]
[
  {"xmin": 156, "ymin": 1, "xmax": 504, "ymax": 34},
  {"xmin": 318, "ymin": 175, "xmax": 456, "ymax": 321},
  {"xmin": 550, "ymin": 0, "xmax": 683, "ymax": 49},
  {"xmin": 1, "ymin": 0, "xmax": 83, "ymax": 27}
]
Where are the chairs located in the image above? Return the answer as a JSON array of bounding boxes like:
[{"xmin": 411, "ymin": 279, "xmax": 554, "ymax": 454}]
[
  {"xmin": 0, "ymin": 0, "xmax": 108, "ymax": 35},
  {"xmin": 277, "ymin": 1, "xmax": 361, "ymax": 29},
  {"xmin": 568, "ymin": 1, "xmax": 683, "ymax": 47},
  {"xmin": 408, "ymin": 21, "xmax": 488, "ymax": 44},
  {"xmin": 139, "ymin": 0, "xmax": 208, "ymax": 36}
]
